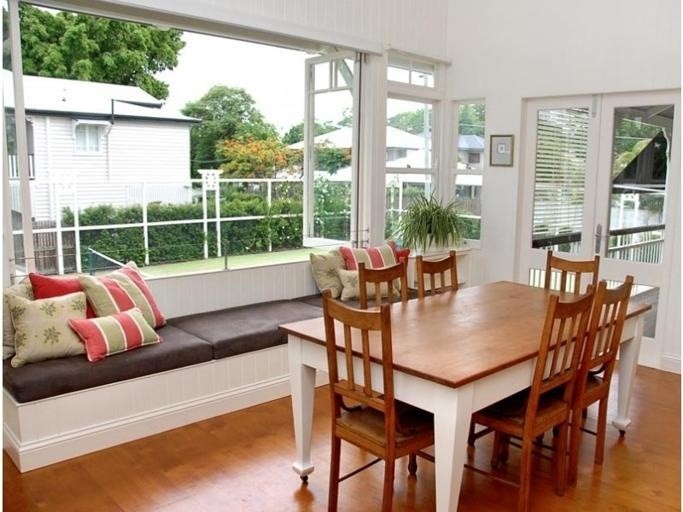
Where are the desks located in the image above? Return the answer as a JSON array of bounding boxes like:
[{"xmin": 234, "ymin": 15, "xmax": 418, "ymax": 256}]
[{"xmin": 277, "ymin": 276, "xmax": 655, "ymax": 511}]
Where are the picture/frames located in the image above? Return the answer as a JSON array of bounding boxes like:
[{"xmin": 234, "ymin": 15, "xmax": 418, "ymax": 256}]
[{"xmin": 486, "ymin": 132, "xmax": 517, "ymax": 169}]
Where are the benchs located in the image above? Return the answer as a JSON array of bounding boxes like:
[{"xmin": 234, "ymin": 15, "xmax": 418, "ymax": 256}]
[{"xmin": 4, "ymin": 245, "xmax": 443, "ymax": 478}]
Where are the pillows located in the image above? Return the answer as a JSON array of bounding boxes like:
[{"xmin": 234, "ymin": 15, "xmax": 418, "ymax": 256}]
[
  {"xmin": 307, "ymin": 238, "xmax": 414, "ymax": 305},
  {"xmin": 2, "ymin": 266, "xmax": 176, "ymax": 366}
]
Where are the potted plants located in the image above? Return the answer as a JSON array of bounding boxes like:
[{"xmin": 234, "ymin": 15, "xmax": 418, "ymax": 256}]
[{"xmin": 397, "ymin": 194, "xmax": 466, "ymax": 251}]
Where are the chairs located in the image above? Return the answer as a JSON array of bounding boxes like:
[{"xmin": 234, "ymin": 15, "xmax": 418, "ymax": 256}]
[
  {"xmin": 502, "ymin": 276, "xmax": 633, "ymax": 488},
  {"xmin": 415, "ymin": 246, "xmax": 460, "ymax": 303},
  {"xmin": 355, "ymin": 257, "xmax": 409, "ymax": 313},
  {"xmin": 317, "ymin": 283, "xmax": 445, "ymax": 512},
  {"xmin": 467, "ymin": 284, "xmax": 595, "ymax": 511},
  {"xmin": 541, "ymin": 250, "xmax": 601, "ymax": 290}
]
